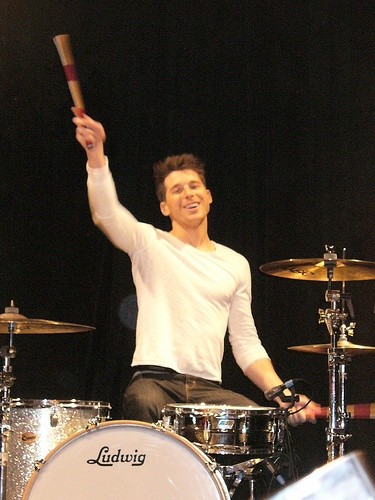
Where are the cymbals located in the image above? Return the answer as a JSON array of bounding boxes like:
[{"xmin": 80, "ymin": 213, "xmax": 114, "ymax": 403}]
[
  {"xmin": 286, "ymin": 342, "xmax": 375, "ymax": 356},
  {"xmin": 258, "ymin": 259, "xmax": 375, "ymax": 281},
  {"xmin": 0, "ymin": 313, "xmax": 95, "ymax": 334}
]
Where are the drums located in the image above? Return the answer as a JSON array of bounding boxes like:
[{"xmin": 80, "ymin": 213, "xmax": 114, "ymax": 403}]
[
  {"xmin": 159, "ymin": 405, "xmax": 289, "ymax": 455},
  {"xmin": 19, "ymin": 420, "xmax": 229, "ymax": 500},
  {"xmin": 0, "ymin": 399, "xmax": 111, "ymax": 500},
  {"xmin": 262, "ymin": 451, "xmax": 375, "ymax": 500}
]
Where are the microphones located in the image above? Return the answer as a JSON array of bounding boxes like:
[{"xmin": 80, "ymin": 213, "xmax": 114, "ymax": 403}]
[{"xmin": 264, "ymin": 379, "xmax": 297, "ymax": 401}]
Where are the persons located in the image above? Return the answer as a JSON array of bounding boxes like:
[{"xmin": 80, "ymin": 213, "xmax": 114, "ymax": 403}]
[{"xmin": 70, "ymin": 106, "xmax": 322, "ymax": 427}]
